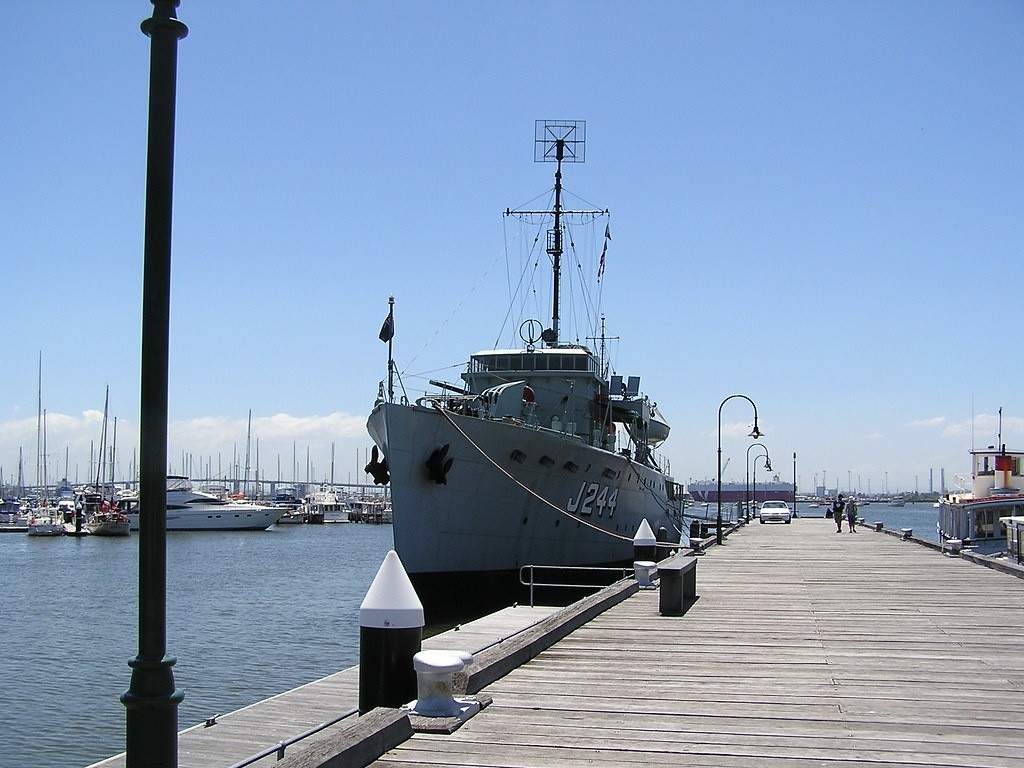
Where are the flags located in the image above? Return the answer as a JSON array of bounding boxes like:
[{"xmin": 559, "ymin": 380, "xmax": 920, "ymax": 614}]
[{"xmin": 379, "ymin": 312, "xmax": 394, "ymax": 343}]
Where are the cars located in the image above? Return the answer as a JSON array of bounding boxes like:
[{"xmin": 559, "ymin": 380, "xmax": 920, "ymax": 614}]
[{"xmin": 758, "ymin": 499, "xmax": 792, "ymax": 527}]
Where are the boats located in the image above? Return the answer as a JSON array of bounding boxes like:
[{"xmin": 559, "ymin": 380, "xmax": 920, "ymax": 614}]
[
  {"xmin": 124, "ymin": 488, "xmax": 289, "ymax": 530},
  {"xmin": 934, "ymin": 406, "xmax": 1024, "ymax": 566},
  {"xmin": 856, "ymin": 501, "xmax": 869, "ymax": 507},
  {"xmin": 683, "ymin": 499, "xmax": 761, "ymax": 509},
  {"xmin": 808, "ymin": 503, "xmax": 820, "ymax": 506},
  {"xmin": 366, "ymin": 120, "xmax": 688, "ymax": 629},
  {"xmin": 888, "ymin": 496, "xmax": 905, "ymax": 507},
  {"xmin": 689, "ymin": 480, "xmax": 796, "ymax": 503}
]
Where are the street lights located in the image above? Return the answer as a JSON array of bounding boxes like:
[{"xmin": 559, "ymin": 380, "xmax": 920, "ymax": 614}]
[
  {"xmin": 745, "ymin": 443, "xmax": 775, "ymax": 523},
  {"xmin": 792, "ymin": 453, "xmax": 798, "ymax": 520},
  {"xmin": 717, "ymin": 395, "xmax": 764, "ymax": 547}
]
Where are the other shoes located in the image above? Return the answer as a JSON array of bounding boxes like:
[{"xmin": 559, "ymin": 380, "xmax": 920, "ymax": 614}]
[
  {"xmin": 849, "ymin": 531, "xmax": 852, "ymax": 533},
  {"xmin": 837, "ymin": 530, "xmax": 842, "ymax": 532},
  {"xmin": 853, "ymin": 530, "xmax": 856, "ymax": 532}
]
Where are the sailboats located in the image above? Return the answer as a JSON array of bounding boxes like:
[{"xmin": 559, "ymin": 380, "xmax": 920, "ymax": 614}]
[{"xmin": 0, "ymin": 347, "xmax": 392, "ymax": 537}]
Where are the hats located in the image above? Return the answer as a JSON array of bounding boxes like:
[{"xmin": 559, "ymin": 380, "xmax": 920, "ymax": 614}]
[{"xmin": 838, "ymin": 494, "xmax": 844, "ymax": 498}]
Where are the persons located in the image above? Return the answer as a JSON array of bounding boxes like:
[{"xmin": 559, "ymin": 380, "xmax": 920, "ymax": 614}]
[
  {"xmin": 846, "ymin": 496, "xmax": 856, "ymax": 533},
  {"xmin": 832, "ymin": 494, "xmax": 845, "ymax": 533}
]
